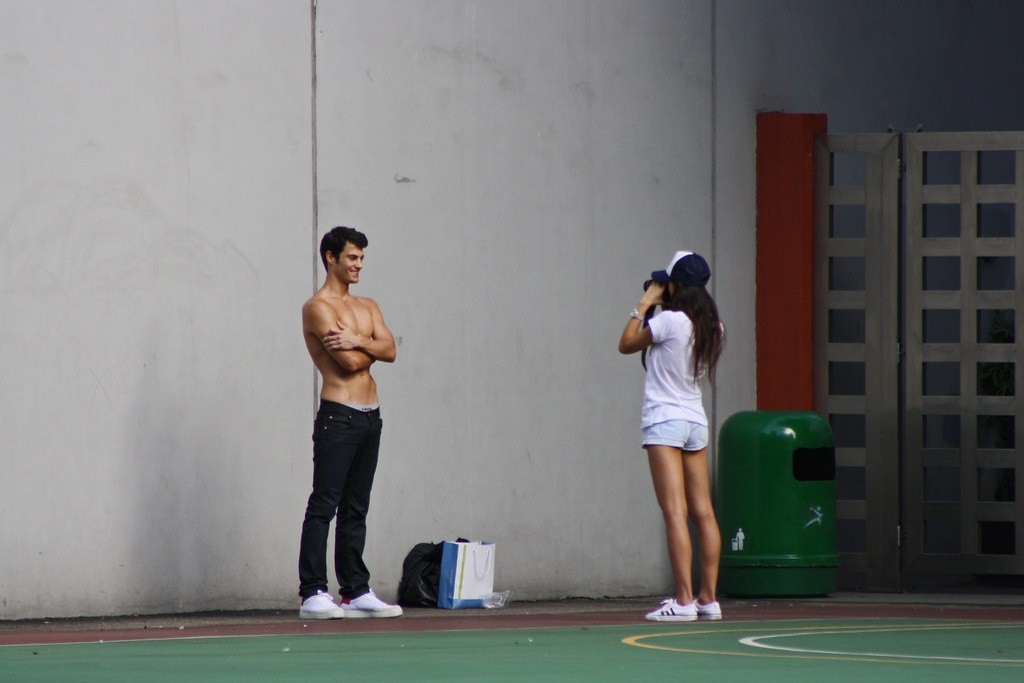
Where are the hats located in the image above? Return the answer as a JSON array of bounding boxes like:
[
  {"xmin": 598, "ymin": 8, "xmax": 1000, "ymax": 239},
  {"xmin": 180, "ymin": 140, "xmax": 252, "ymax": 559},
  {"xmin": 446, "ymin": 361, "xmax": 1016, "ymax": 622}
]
[{"xmin": 652, "ymin": 251, "xmax": 711, "ymax": 286}]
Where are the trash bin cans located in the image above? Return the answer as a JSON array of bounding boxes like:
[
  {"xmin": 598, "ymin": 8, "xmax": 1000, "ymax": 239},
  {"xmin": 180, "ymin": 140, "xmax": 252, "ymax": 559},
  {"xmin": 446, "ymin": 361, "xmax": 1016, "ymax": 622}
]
[{"xmin": 717, "ymin": 410, "xmax": 843, "ymax": 597}]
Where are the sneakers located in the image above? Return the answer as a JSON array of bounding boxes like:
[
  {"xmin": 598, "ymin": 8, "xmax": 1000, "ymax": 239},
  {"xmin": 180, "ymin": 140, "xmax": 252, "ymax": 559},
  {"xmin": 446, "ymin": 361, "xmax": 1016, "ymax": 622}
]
[
  {"xmin": 645, "ymin": 599, "xmax": 699, "ymax": 622},
  {"xmin": 340, "ymin": 588, "xmax": 403, "ymax": 619},
  {"xmin": 694, "ymin": 599, "xmax": 722, "ymax": 621},
  {"xmin": 298, "ymin": 589, "xmax": 345, "ymax": 620}
]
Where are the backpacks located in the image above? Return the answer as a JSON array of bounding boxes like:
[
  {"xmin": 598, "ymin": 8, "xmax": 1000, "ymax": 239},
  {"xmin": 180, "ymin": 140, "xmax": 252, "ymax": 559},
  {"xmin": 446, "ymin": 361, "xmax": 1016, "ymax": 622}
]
[{"xmin": 396, "ymin": 538, "xmax": 470, "ymax": 608}]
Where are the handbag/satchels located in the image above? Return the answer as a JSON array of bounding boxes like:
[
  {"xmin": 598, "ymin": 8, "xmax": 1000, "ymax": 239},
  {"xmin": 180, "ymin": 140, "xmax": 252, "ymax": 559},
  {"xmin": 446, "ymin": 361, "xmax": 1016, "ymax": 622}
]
[{"xmin": 437, "ymin": 540, "xmax": 496, "ymax": 610}]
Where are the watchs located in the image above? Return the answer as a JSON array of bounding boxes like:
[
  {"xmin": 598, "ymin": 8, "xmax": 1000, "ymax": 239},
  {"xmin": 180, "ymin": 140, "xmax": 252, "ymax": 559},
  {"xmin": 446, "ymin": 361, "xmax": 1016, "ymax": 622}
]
[{"xmin": 630, "ymin": 309, "xmax": 644, "ymax": 321}]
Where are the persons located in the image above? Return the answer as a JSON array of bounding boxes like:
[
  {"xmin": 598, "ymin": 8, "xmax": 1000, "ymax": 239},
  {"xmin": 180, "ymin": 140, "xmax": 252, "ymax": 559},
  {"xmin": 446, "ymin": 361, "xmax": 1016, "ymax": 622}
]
[
  {"xmin": 298, "ymin": 226, "xmax": 403, "ymax": 620},
  {"xmin": 619, "ymin": 251, "xmax": 724, "ymax": 622}
]
